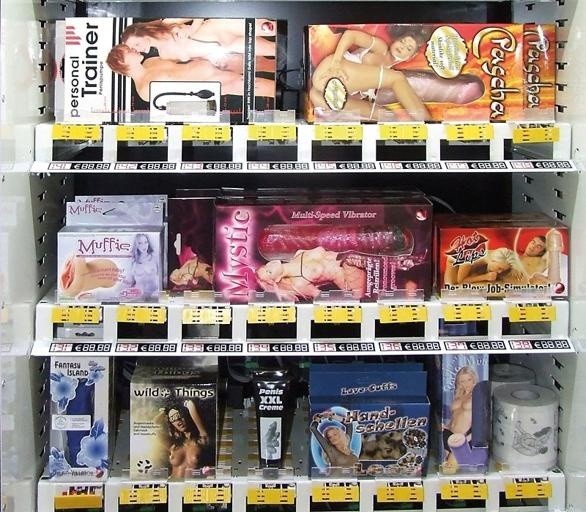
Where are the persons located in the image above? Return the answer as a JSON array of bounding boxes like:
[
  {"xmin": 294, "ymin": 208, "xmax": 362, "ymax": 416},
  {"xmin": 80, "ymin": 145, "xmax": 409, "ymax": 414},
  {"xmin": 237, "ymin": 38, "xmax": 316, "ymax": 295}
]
[
  {"xmin": 311, "ymin": 411, "xmax": 359, "ymax": 479},
  {"xmin": 254, "ymin": 244, "xmax": 367, "ymax": 302},
  {"xmin": 442, "ymin": 365, "xmax": 480, "ymax": 452},
  {"xmin": 104, "ymin": 45, "xmax": 276, "ymax": 105},
  {"xmin": 118, "ymin": 234, "xmax": 158, "ymax": 300},
  {"xmin": 444, "ymin": 246, "xmax": 523, "ymax": 287},
  {"xmin": 149, "ymin": 398, "xmax": 210, "ymax": 481},
  {"xmin": 120, "ymin": 17, "xmax": 277, "ymax": 64},
  {"xmin": 463, "ymin": 236, "xmax": 547, "ymax": 289},
  {"xmin": 169, "ymin": 258, "xmax": 212, "ymax": 286},
  {"xmin": 309, "ymin": 26, "xmax": 434, "ymax": 122}
]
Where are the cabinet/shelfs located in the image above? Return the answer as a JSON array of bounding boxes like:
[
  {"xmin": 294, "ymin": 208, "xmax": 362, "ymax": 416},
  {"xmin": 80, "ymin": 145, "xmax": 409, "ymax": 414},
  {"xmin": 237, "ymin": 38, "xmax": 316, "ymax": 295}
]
[{"xmin": 0, "ymin": 1, "xmax": 584, "ymax": 512}]
